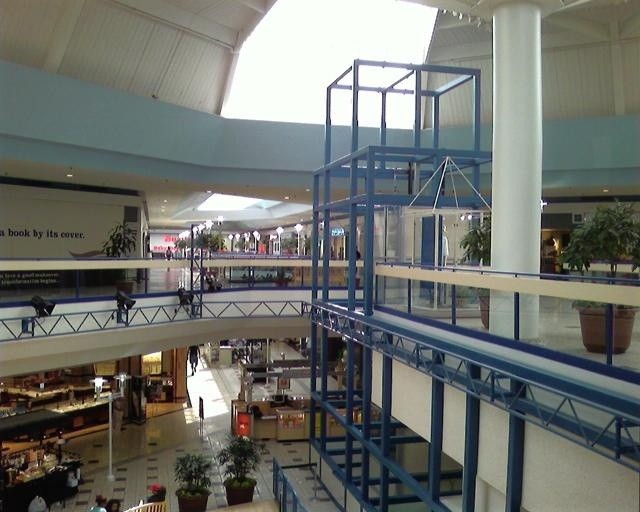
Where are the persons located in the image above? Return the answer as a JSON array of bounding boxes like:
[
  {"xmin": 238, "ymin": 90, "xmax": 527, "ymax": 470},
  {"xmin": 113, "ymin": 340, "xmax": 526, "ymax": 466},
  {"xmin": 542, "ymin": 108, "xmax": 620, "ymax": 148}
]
[
  {"xmin": 443, "ymin": 226, "xmax": 450, "ymax": 265},
  {"xmin": 87, "ymin": 493, "xmax": 107, "ymax": 511},
  {"xmin": 105, "ymin": 499, "xmax": 121, "ymax": 512},
  {"xmin": 165, "ymin": 245, "xmax": 172, "ymax": 261},
  {"xmin": 186, "ymin": 344, "xmax": 201, "ymax": 377}
]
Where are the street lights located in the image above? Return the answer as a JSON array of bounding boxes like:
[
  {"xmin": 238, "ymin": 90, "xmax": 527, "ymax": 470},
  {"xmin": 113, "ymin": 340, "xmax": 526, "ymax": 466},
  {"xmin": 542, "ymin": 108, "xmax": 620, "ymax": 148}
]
[
  {"xmin": 91, "ymin": 370, "xmax": 131, "ymax": 481},
  {"xmin": 217, "ymin": 215, "xmax": 224, "ymax": 254},
  {"xmin": 179, "ymin": 218, "xmax": 214, "ymax": 260},
  {"xmin": 228, "ymin": 232, "xmax": 251, "ymax": 253},
  {"xmin": 295, "ymin": 223, "xmax": 303, "ymax": 256},
  {"xmin": 253, "ymin": 230, "xmax": 260, "ymax": 252},
  {"xmin": 275, "ymin": 227, "xmax": 285, "ymax": 256}
]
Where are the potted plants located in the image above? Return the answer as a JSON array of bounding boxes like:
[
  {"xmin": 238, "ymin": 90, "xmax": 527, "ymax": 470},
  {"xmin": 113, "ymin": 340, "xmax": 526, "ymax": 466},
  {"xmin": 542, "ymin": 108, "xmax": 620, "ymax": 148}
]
[
  {"xmin": 102, "ymin": 223, "xmax": 138, "ymax": 294},
  {"xmin": 554, "ymin": 199, "xmax": 640, "ymax": 354},
  {"xmin": 173, "ymin": 454, "xmax": 213, "ymax": 512},
  {"xmin": 213, "ymin": 433, "xmax": 269, "ymax": 506},
  {"xmin": 458, "ymin": 215, "xmax": 491, "ymax": 329}
]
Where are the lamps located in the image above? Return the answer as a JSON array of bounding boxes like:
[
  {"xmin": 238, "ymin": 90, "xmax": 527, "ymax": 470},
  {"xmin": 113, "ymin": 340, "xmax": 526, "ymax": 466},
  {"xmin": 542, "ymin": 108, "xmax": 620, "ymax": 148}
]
[
  {"xmin": 112, "ymin": 289, "xmax": 136, "ymax": 323},
  {"xmin": 177, "ymin": 287, "xmax": 194, "ymax": 311},
  {"xmin": 22, "ymin": 295, "xmax": 57, "ymax": 334}
]
